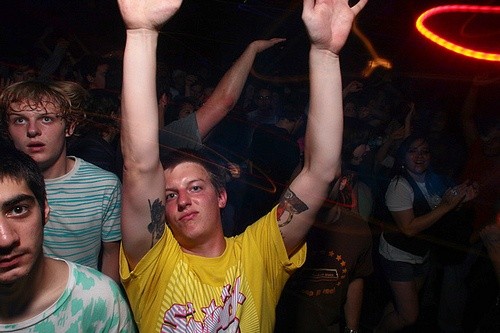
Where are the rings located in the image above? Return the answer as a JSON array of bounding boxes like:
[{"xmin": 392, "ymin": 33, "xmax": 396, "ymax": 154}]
[{"xmin": 451, "ymin": 190, "xmax": 458, "ymax": 195}]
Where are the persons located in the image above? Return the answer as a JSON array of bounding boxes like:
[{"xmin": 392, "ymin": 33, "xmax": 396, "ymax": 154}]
[
  {"xmin": 374, "ymin": 137, "xmax": 481, "ymax": 333},
  {"xmin": 275, "ymin": 161, "xmax": 366, "ymax": 333},
  {"xmin": 117, "ymin": 0, "xmax": 370, "ymax": 333},
  {"xmin": 70, "ymin": 36, "xmax": 500, "ymax": 197},
  {"xmin": 0, "ymin": 75, "xmax": 122, "ymax": 285},
  {"xmin": 0, "ymin": 149, "xmax": 139, "ymax": 333}
]
